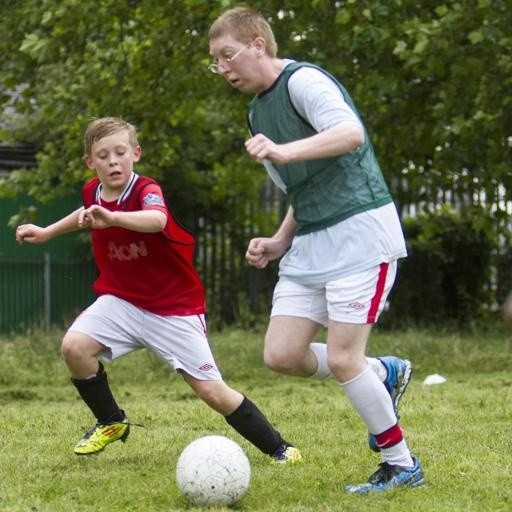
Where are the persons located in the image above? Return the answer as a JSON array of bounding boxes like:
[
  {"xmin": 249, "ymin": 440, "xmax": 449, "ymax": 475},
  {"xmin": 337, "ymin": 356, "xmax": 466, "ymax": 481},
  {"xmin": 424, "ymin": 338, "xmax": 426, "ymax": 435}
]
[
  {"xmin": 14, "ymin": 118, "xmax": 303, "ymax": 464},
  {"xmin": 206, "ymin": 7, "xmax": 429, "ymax": 499}
]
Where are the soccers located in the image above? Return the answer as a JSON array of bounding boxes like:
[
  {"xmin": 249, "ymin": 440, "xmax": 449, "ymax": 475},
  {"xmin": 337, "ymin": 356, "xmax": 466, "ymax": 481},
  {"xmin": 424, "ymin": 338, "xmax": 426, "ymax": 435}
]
[{"xmin": 177, "ymin": 435, "xmax": 250, "ymax": 507}]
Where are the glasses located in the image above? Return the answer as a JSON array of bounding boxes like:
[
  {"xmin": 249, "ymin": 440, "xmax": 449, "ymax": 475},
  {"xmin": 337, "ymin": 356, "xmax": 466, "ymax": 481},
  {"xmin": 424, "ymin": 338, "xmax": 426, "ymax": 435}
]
[{"xmin": 208, "ymin": 43, "xmax": 252, "ymax": 73}]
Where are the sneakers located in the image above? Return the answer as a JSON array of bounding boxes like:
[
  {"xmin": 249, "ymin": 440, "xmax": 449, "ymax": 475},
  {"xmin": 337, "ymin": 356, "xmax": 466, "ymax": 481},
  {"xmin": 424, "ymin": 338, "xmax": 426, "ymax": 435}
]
[
  {"xmin": 369, "ymin": 355, "xmax": 412, "ymax": 453},
  {"xmin": 266, "ymin": 441, "xmax": 303, "ymax": 464},
  {"xmin": 74, "ymin": 409, "xmax": 131, "ymax": 456},
  {"xmin": 346, "ymin": 456, "xmax": 425, "ymax": 495}
]
